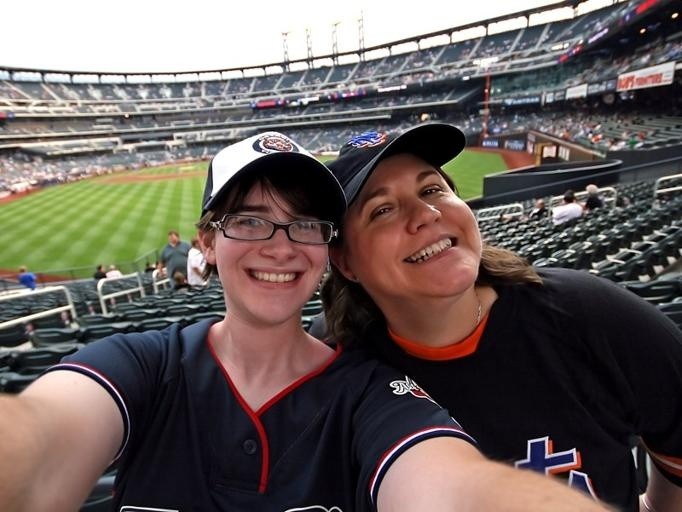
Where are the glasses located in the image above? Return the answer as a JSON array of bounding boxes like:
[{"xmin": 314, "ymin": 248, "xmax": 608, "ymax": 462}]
[{"xmin": 209, "ymin": 214, "xmax": 341, "ymax": 245}]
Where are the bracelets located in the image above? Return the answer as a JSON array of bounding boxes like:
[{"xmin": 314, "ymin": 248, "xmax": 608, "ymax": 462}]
[{"xmin": 641, "ymin": 490, "xmax": 651, "ymax": 511}]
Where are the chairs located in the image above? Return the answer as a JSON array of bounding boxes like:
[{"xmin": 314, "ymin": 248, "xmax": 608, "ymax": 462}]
[
  {"xmin": 0, "ymin": 274, "xmax": 226, "ymax": 397},
  {"xmin": 475, "ymin": 177, "xmax": 682, "ymax": 326}
]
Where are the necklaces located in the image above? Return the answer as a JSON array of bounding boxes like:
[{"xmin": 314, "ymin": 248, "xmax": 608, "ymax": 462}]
[{"xmin": 470, "ymin": 288, "xmax": 486, "ymax": 328}]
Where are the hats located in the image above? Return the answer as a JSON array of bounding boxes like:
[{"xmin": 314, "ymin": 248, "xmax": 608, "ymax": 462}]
[
  {"xmin": 323, "ymin": 123, "xmax": 466, "ymax": 206},
  {"xmin": 202, "ymin": 131, "xmax": 348, "ymax": 226}
]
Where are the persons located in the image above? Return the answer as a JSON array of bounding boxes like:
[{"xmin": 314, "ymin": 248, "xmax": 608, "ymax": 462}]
[
  {"xmin": 0, "ymin": 131, "xmax": 612, "ymax": 511},
  {"xmin": 17, "ymin": 264, "xmax": 37, "ymax": 290},
  {"xmin": 548, "ymin": 191, "xmax": 584, "ymax": 225},
  {"xmin": 496, "ymin": 198, "xmax": 552, "ymax": 225},
  {"xmin": 103, "ymin": 264, "xmax": 123, "ymax": 280},
  {"xmin": 145, "ymin": 230, "xmax": 214, "ymax": 292},
  {"xmin": 302, "ymin": 122, "xmax": 682, "ymax": 511},
  {"xmin": 92, "ymin": 262, "xmax": 106, "ymax": 279},
  {"xmin": 1, "ymin": 1, "xmax": 682, "ymax": 214}
]
